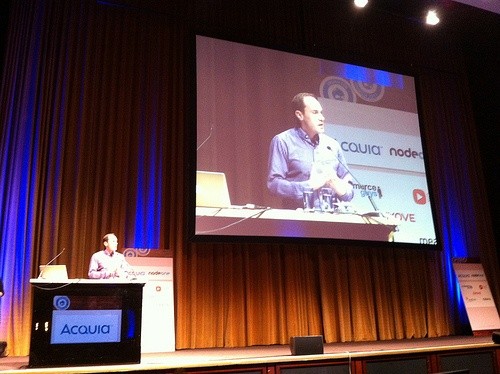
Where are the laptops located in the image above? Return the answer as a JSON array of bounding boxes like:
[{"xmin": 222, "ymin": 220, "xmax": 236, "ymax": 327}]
[
  {"xmin": 196, "ymin": 171, "xmax": 232, "ymax": 208},
  {"xmin": 39, "ymin": 264, "xmax": 69, "ymax": 279}
]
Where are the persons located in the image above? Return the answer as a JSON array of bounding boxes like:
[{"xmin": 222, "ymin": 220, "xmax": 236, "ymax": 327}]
[
  {"xmin": 88, "ymin": 234, "xmax": 136, "ymax": 278},
  {"xmin": 266, "ymin": 92, "xmax": 354, "ymax": 213}
]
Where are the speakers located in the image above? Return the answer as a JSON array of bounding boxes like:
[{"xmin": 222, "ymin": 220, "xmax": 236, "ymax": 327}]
[
  {"xmin": 289, "ymin": 335, "xmax": 324, "ymax": 355},
  {"xmin": 492, "ymin": 332, "xmax": 500, "ymax": 344}
]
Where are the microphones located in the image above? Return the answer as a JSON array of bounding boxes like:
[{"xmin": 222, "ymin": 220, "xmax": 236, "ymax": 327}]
[
  {"xmin": 37, "ymin": 248, "xmax": 66, "ymax": 279},
  {"xmin": 197, "ymin": 127, "xmax": 213, "ymax": 150},
  {"xmin": 117, "ymin": 254, "xmax": 137, "ymax": 280},
  {"xmin": 327, "ymin": 146, "xmax": 381, "ymax": 216}
]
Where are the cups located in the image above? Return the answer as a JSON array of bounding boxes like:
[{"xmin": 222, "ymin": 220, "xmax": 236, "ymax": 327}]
[
  {"xmin": 101, "ymin": 269, "xmax": 106, "ymax": 279},
  {"xmin": 321, "ymin": 188, "xmax": 333, "ymax": 213},
  {"xmin": 110, "ymin": 269, "xmax": 115, "ymax": 280},
  {"xmin": 303, "ymin": 187, "xmax": 314, "ymax": 213}
]
